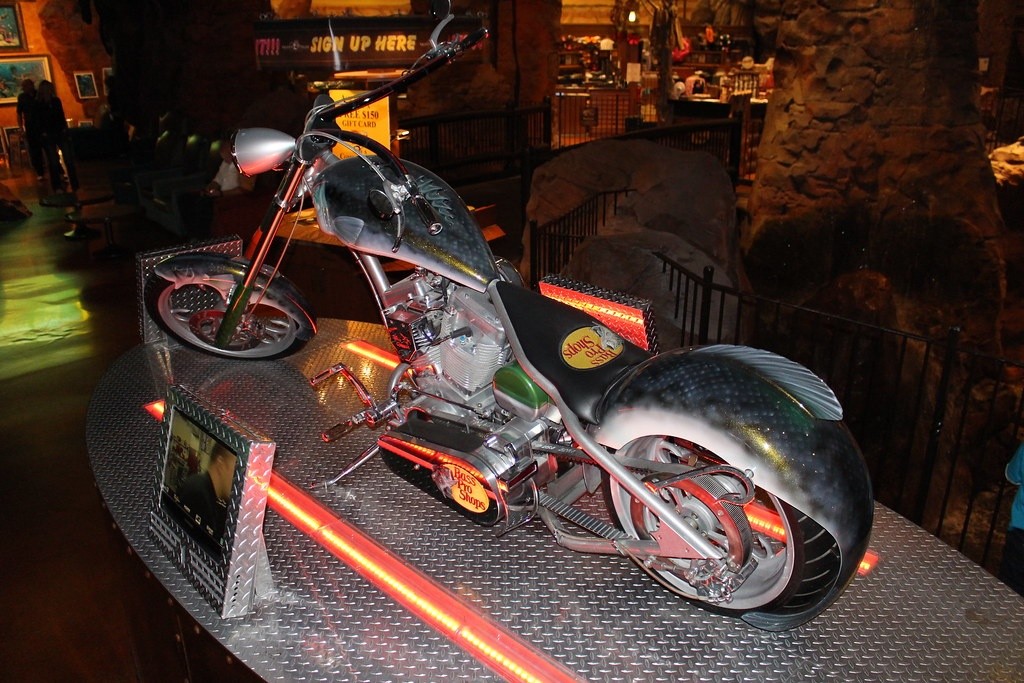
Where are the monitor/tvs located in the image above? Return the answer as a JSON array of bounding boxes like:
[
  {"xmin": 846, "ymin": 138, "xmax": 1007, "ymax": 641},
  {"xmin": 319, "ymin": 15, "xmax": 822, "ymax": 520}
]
[{"xmin": 162, "ymin": 408, "xmax": 237, "ymax": 564}]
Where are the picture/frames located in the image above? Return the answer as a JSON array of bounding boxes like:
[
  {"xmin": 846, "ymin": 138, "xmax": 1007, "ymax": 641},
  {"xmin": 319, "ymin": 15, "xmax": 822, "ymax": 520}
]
[
  {"xmin": 0, "ymin": 1, "xmax": 28, "ymax": 53},
  {"xmin": 0, "ymin": 55, "xmax": 53, "ymax": 105},
  {"xmin": 103, "ymin": 68, "xmax": 112, "ymax": 95},
  {"xmin": 75, "ymin": 72, "xmax": 99, "ymax": 99}
]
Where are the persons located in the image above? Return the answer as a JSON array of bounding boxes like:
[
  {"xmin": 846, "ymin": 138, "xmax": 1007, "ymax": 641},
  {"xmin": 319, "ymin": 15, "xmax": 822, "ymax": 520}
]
[
  {"xmin": 16, "ymin": 79, "xmax": 68, "ymax": 181},
  {"xmin": 180, "ymin": 141, "xmax": 256, "ymax": 239},
  {"xmin": 28, "ymin": 81, "xmax": 80, "ymax": 194}
]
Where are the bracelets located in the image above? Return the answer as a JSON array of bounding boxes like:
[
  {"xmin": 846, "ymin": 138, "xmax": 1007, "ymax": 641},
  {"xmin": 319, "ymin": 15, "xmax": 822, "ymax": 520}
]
[{"xmin": 220, "ymin": 191, "xmax": 223, "ymax": 197}]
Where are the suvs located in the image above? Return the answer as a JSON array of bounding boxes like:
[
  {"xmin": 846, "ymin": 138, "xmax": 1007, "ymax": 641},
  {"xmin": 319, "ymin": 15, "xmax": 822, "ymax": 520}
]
[{"xmin": 559, "ymin": 51, "xmax": 586, "ymax": 86}]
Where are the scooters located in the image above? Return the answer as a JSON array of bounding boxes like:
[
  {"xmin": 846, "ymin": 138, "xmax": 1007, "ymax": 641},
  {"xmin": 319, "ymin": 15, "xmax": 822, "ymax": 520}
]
[{"xmin": 143, "ymin": 13, "xmax": 870, "ymax": 630}]
